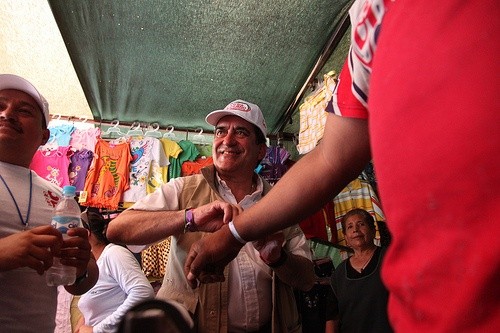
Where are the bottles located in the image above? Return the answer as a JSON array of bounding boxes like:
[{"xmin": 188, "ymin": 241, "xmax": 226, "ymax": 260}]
[{"xmin": 46, "ymin": 186, "xmax": 81, "ymax": 286}]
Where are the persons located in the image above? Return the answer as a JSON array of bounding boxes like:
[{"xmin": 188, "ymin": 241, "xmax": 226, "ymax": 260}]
[
  {"xmin": 106, "ymin": 99, "xmax": 316, "ymax": 333},
  {"xmin": 0, "ymin": 73, "xmax": 99, "ymax": 333},
  {"xmin": 184, "ymin": 0, "xmax": 500, "ymax": 333},
  {"xmin": 324, "ymin": 209, "xmax": 390, "ymax": 333},
  {"xmin": 74, "ymin": 207, "xmax": 154, "ymax": 333}
]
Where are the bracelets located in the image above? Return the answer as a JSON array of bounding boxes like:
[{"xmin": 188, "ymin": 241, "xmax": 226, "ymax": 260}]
[
  {"xmin": 74, "ymin": 272, "xmax": 88, "ymax": 289},
  {"xmin": 259, "ymin": 249, "xmax": 287, "ymax": 268},
  {"xmin": 228, "ymin": 221, "xmax": 247, "ymax": 245},
  {"xmin": 184, "ymin": 206, "xmax": 194, "ymax": 234}
]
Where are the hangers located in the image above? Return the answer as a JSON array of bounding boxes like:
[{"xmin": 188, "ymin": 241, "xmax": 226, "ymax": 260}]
[
  {"xmin": 127, "ymin": 121, "xmax": 144, "ymax": 137},
  {"xmin": 102, "ymin": 120, "xmax": 126, "ymax": 137},
  {"xmin": 71, "ymin": 134, "xmax": 83, "ymax": 150},
  {"xmin": 40, "ymin": 136, "xmax": 58, "ymax": 151},
  {"xmin": 69, "ymin": 115, "xmax": 95, "ymax": 131},
  {"xmin": 191, "ymin": 127, "xmax": 212, "ymax": 155},
  {"xmin": 164, "ymin": 125, "xmax": 182, "ymax": 143},
  {"xmin": 145, "ymin": 123, "xmax": 162, "ymax": 139},
  {"xmin": 48, "ymin": 113, "xmax": 68, "ymax": 127}
]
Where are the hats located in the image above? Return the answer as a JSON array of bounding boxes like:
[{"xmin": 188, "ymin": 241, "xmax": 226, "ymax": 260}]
[
  {"xmin": 0, "ymin": 74, "xmax": 49, "ymax": 127},
  {"xmin": 205, "ymin": 100, "xmax": 267, "ymax": 142}
]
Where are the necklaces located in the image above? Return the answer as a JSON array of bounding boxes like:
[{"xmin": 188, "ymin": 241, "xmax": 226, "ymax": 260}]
[{"xmin": 0, "ymin": 170, "xmax": 32, "ymax": 230}]
